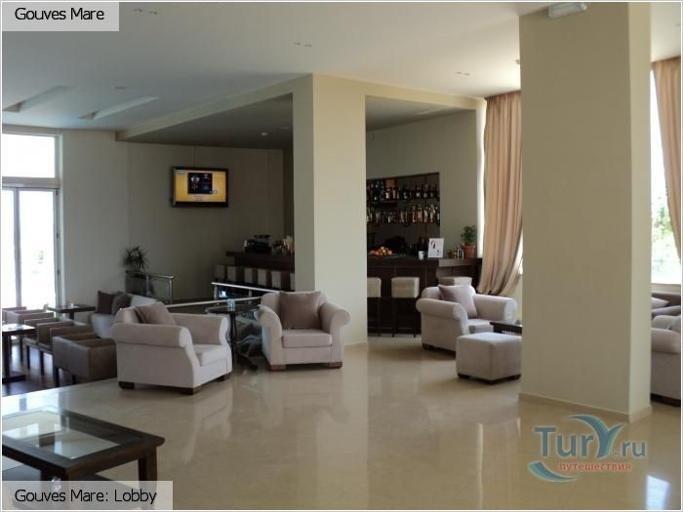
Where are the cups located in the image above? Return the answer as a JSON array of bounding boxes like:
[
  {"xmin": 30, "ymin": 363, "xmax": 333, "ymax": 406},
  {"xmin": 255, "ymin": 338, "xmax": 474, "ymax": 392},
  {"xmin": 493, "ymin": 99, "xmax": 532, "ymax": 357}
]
[{"xmin": 417, "ymin": 251, "xmax": 425, "ymax": 261}]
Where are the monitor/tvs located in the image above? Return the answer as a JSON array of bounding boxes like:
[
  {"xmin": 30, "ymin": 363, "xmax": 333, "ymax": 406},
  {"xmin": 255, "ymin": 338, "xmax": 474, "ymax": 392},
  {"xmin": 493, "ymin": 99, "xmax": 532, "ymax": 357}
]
[{"xmin": 170, "ymin": 166, "xmax": 229, "ymax": 208}]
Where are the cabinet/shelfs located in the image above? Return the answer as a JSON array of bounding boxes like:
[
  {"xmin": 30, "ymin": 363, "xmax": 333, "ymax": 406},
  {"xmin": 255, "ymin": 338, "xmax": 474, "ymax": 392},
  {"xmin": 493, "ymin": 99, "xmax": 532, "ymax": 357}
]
[{"xmin": 366, "ymin": 189, "xmax": 438, "ymax": 227}]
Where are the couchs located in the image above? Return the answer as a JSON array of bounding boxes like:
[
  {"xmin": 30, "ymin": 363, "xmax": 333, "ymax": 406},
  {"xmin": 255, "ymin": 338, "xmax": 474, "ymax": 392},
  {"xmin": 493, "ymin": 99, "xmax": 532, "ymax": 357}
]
[
  {"xmin": 454, "ymin": 331, "xmax": 522, "ymax": 385},
  {"xmin": 417, "ymin": 285, "xmax": 520, "ymax": 358},
  {"xmin": 90, "ymin": 291, "xmax": 158, "ymax": 339},
  {"xmin": 650, "ymin": 314, "xmax": 680, "ymax": 409},
  {"xmin": 651, "ymin": 291, "xmax": 680, "ymax": 317},
  {"xmin": 1, "ymin": 300, "xmax": 114, "ymax": 393},
  {"xmin": 113, "ymin": 297, "xmax": 233, "ymax": 396},
  {"xmin": 257, "ymin": 283, "xmax": 354, "ymax": 373}
]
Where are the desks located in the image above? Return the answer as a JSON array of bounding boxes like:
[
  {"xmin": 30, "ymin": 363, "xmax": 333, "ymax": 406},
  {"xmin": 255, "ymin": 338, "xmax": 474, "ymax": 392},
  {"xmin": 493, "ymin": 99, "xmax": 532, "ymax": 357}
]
[{"xmin": 2, "ymin": 406, "xmax": 165, "ymax": 510}]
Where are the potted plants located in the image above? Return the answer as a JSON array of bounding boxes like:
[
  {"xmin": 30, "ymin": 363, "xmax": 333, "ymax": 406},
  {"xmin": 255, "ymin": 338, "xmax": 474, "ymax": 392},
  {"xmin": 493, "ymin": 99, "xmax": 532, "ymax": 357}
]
[
  {"xmin": 125, "ymin": 247, "xmax": 150, "ymax": 297},
  {"xmin": 462, "ymin": 224, "xmax": 477, "ymax": 258}
]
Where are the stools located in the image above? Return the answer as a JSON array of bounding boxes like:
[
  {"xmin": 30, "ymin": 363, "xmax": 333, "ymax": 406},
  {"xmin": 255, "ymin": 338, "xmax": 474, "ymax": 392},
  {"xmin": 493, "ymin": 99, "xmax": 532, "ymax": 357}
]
[
  {"xmin": 437, "ymin": 274, "xmax": 473, "ymax": 285},
  {"xmin": 214, "ymin": 263, "xmax": 295, "ymax": 294},
  {"xmin": 389, "ymin": 275, "xmax": 418, "ymax": 338},
  {"xmin": 364, "ymin": 277, "xmax": 382, "ymax": 336}
]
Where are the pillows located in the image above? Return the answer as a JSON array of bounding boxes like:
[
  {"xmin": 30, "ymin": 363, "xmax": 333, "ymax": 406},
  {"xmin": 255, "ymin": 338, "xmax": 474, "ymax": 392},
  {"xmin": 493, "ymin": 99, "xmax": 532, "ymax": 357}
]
[
  {"xmin": 135, "ymin": 301, "xmax": 179, "ymax": 329},
  {"xmin": 110, "ymin": 294, "xmax": 133, "ymax": 316},
  {"xmin": 96, "ymin": 291, "xmax": 113, "ymax": 314},
  {"xmin": 275, "ymin": 287, "xmax": 324, "ymax": 330},
  {"xmin": 438, "ymin": 285, "xmax": 478, "ymax": 320},
  {"xmin": 651, "ymin": 296, "xmax": 669, "ymax": 309}
]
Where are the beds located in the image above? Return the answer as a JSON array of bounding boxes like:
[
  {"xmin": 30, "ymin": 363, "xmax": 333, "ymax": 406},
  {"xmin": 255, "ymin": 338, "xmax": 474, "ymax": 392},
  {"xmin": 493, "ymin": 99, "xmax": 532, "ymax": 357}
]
[{"xmin": 490, "ymin": 314, "xmax": 521, "ymax": 337}]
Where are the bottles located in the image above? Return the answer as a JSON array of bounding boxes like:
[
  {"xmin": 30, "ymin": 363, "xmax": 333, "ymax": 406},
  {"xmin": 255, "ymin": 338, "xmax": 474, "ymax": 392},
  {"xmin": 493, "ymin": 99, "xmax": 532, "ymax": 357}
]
[
  {"xmin": 366, "ymin": 181, "xmax": 439, "ymax": 225},
  {"xmin": 270, "ymin": 236, "xmax": 293, "ymax": 256},
  {"xmin": 446, "ymin": 246, "xmax": 464, "ymax": 260}
]
